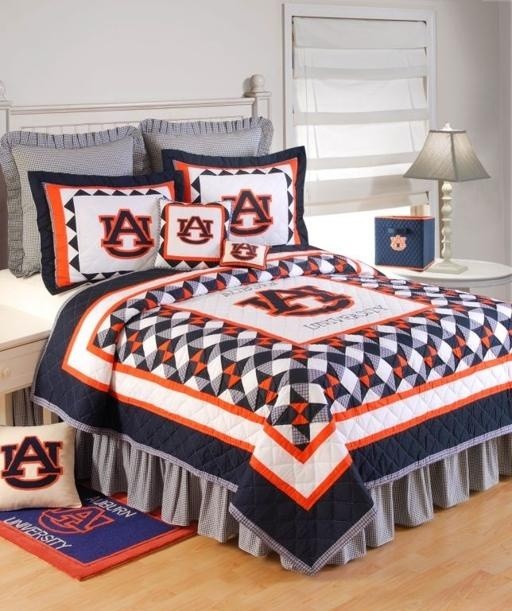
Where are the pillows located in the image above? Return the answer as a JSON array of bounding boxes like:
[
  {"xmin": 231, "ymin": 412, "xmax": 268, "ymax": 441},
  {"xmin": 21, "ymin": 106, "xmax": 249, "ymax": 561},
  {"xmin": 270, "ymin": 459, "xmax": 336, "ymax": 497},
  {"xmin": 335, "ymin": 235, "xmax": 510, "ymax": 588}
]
[
  {"xmin": 138, "ymin": 110, "xmax": 277, "ymax": 173},
  {"xmin": 155, "ymin": 197, "xmax": 235, "ymax": 271},
  {"xmin": 220, "ymin": 237, "xmax": 271, "ymax": 271},
  {"xmin": 159, "ymin": 143, "xmax": 311, "ymax": 253},
  {"xmin": 0, "ymin": 418, "xmax": 82, "ymax": 513},
  {"xmin": 0, "ymin": 125, "xmax": 147, "ymax": 280},
  {"xmin": 23, "ymin": 162, "xmax": 188, "ymax": 295}
]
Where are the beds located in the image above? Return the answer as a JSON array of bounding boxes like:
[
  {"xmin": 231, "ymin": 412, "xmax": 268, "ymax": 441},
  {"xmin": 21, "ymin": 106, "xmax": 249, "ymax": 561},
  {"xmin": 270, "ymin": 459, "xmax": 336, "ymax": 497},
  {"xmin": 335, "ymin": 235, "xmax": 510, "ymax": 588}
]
[{"xmin": 0, "ymin": 67, "xmax": 511, "ymax": 574}]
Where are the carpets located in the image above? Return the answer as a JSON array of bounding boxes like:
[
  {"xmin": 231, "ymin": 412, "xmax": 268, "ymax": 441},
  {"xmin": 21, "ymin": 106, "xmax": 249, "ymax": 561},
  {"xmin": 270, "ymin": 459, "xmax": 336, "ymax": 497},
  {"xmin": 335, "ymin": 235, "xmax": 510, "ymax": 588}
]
[{"xmin": 0, "ymin": 485, "xmax": 201, "ymax": 582}]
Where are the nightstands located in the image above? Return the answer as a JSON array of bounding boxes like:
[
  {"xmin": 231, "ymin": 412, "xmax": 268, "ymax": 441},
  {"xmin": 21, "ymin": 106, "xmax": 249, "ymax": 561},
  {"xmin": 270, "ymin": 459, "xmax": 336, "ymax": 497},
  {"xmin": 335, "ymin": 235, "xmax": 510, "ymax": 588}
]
[{"xmin": 0, "ymin": 284, "xmax": 67, "ymax": 426}]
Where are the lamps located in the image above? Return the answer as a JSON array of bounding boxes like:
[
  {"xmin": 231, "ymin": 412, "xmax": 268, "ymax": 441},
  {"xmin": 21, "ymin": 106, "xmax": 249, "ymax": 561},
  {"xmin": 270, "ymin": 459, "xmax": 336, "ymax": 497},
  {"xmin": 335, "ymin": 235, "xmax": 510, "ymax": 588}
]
[{"xmin": 401, "ymin": 124, "xmax": 491, "ymax": 274}]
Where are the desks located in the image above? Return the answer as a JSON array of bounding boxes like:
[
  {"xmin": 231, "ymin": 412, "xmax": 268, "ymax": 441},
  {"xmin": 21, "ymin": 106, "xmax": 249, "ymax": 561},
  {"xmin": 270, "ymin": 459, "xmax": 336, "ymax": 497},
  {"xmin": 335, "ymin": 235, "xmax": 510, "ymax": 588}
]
[{"xmin": 391, "ymin": 259, "xmax": 512, "ymax": 291}]
